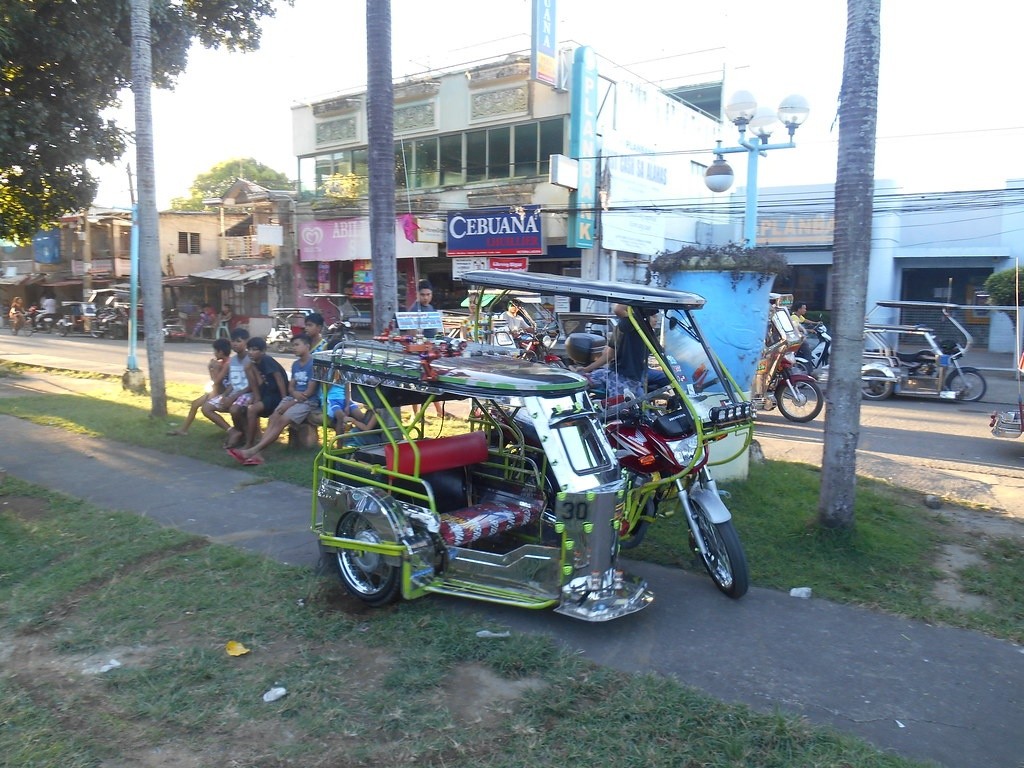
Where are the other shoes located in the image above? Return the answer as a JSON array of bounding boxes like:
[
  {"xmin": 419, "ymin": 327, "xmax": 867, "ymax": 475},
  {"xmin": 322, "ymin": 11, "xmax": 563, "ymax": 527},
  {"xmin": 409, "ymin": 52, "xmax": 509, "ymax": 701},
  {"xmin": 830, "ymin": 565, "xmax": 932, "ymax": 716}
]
[
  {"xmin": 223, "ymin": 429, "xmax": 243, "ymax": 448},
  {"xmin": 166, "ymin": 429, "xmax": 189, "ymax": 436}
]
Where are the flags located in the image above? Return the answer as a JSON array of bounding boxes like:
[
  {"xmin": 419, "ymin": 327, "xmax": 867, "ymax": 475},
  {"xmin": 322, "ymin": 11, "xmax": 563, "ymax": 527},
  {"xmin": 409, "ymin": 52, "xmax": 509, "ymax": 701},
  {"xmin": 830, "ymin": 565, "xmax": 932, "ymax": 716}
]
[{"xmin": 403, "ymin": 214, "xmax": 419, "ymax": 243}]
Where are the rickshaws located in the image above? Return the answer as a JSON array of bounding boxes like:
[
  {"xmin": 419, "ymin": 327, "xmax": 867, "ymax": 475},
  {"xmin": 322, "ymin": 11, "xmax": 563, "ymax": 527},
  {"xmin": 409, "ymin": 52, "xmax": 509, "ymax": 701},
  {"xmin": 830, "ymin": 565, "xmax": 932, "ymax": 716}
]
[
  {"xmin": 860, "ymin": 301, "xmax": 987, "ymax": 402},
  {"xmin": 308, "ymin": 269, "xmax": 833, "ymax": 620}
]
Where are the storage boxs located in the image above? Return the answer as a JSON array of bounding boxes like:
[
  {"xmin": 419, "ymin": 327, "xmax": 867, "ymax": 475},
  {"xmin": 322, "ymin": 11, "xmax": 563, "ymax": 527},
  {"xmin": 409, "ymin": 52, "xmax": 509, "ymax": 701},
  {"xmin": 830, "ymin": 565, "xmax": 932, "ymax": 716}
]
[{"xmin": 564, "ymin": 332, "xmax": 606, "ymax": 365}]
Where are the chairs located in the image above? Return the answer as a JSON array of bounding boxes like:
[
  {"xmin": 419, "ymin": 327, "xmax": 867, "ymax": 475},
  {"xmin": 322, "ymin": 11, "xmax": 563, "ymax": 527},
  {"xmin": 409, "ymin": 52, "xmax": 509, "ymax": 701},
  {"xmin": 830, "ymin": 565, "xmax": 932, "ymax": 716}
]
[{"xmin": 201, "ymin": 313, "xmax": 233, "ymax": 342}]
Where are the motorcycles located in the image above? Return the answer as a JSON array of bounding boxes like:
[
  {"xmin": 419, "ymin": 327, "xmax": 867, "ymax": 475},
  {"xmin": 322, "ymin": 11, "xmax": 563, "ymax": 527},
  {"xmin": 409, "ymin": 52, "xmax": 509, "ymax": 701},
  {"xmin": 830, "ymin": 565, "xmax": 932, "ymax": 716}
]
[
  {"xmin": 28, "ymin": 302, "xmax": 187, "ymax": 340},
  {"xmin": 323, "ymin": 320, "xmax": 356, "ymax": 345}
]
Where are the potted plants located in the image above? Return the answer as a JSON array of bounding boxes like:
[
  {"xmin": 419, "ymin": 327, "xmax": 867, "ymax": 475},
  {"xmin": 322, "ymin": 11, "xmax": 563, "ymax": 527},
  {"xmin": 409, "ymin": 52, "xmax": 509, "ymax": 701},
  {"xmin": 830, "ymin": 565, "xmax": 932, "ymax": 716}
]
[{"xmin": 309, "ymin": 173, "xmax": 362, "ymax": 221}]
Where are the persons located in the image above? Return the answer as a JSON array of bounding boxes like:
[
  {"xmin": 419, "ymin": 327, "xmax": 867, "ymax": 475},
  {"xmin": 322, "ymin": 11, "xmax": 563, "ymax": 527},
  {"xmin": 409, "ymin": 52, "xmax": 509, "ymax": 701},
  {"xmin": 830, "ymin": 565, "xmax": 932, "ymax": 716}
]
[
  {"xmin": 790, "ymin": 301, "xmax": 818, "ymax": 380},
  {"xmin": 165, "ymin": 304, "xmax": 381, "ymax": 466},
  {"xmin": 0, "ymin": 294, "xmax": 57, "ymax": 335},
  {"xmin": 460, "ymin": 293, "xmax": 494, "ymax": 345},
  {"xmin": 499, "ymin": 300, "xmax": 533, "ymax": 338},
  {"xmin": 579, "ymin": 303, "xmax": 679, "ymax": 411},
  {"xmin": 772, "ymin": 297, "xmax": 783, "ymax": 307},
  {"xmin": 409, "ymin": 280, "xmax": 436, "ymax": 338}
]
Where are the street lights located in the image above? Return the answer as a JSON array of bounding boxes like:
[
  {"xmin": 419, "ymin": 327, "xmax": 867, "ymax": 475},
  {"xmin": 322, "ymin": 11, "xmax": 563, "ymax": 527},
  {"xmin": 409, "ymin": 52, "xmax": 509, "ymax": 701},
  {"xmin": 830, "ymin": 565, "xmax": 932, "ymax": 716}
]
[{"xmin": 704, "ymin": 89, "xmax": 809, "ymax": 249}]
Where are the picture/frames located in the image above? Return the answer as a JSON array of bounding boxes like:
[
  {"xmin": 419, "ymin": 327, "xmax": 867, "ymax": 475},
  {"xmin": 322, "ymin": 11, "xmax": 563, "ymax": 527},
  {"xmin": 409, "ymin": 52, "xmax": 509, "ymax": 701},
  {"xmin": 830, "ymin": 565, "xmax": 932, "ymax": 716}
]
[{"xmin": 964, "ymin": 283, "xmax": 990, "ymax": 326}]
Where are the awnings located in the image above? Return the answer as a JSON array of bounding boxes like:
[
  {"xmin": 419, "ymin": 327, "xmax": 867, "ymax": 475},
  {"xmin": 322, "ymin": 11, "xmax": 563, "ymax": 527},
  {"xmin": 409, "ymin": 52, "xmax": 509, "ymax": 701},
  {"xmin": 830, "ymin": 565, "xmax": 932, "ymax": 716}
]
[
  {"xmin": 0, "ymin": 272, "xmax": 82, "ymax": 286},
  {"xmin": 162, "ymin": 265, "xmax": 278, "ymax": 293}
]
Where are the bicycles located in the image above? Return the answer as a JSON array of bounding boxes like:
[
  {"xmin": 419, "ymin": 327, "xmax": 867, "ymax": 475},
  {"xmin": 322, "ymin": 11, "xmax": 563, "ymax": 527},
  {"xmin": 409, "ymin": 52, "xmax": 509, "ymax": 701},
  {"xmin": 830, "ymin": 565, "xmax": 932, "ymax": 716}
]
[{"xmin": 10, "ymin": 312, "xmax": 33, "ymax": 337}]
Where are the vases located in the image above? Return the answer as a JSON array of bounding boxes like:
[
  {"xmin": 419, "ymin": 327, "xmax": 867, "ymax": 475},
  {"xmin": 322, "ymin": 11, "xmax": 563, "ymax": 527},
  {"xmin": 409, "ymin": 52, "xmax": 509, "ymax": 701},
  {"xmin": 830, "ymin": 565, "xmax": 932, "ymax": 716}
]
[
  {"xmin": 395, "ymin": 199, "xmax": 439, "ymax": 215},
  {"xmin": 466, "ymin": 188, "xmax": 532, "ymax": 209}
]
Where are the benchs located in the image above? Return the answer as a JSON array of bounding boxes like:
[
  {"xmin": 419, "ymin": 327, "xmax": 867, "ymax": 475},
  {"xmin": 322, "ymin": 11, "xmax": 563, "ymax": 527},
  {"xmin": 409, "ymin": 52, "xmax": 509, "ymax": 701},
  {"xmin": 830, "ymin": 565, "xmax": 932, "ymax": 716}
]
[
  {"xmin": 220, "ymin": 405, "xmax": 353, "ymax": 451},
  {"xmin": 385, "ymin": 429, "xmax": 538, "ymax": 547}
]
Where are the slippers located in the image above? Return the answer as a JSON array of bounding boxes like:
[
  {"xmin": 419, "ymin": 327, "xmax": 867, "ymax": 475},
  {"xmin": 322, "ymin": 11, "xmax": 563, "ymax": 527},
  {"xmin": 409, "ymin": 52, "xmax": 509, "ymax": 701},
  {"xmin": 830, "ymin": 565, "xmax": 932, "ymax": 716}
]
[
  {"xmin": 243, "ymin": 458, "xmax": 263, "ymax": 465},
  {"xmin": 227, "ymin": 448, "xmax": 244, "ymax": 464}
]
